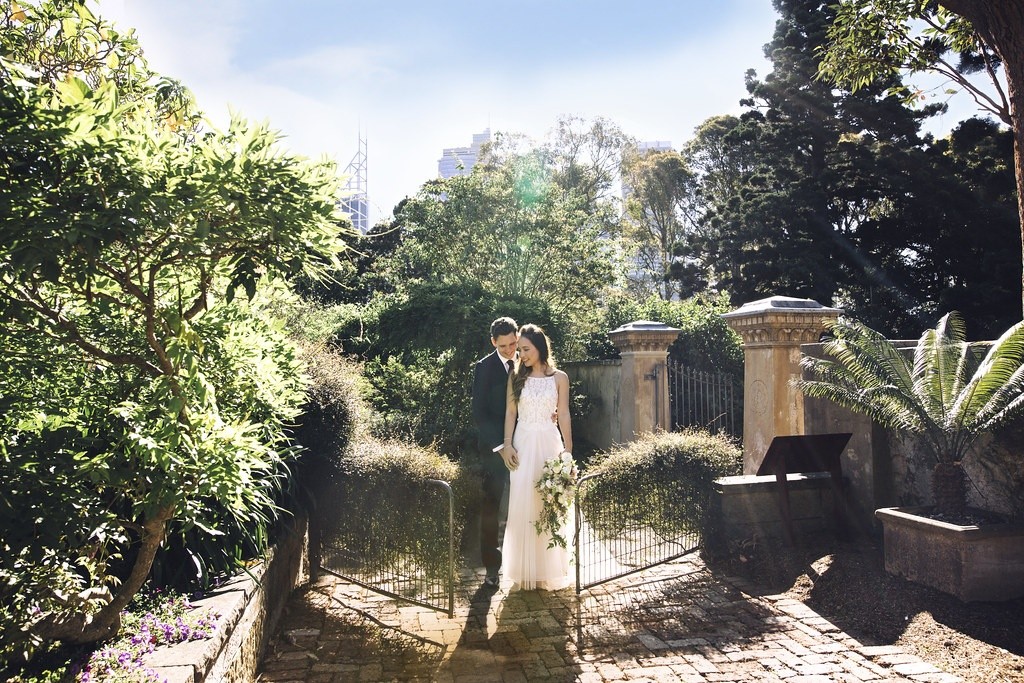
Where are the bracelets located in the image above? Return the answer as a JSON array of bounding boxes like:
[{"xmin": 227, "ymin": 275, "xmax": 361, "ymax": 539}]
[
  {"xmin": 504, "ymin": 445, "xmax": 512, "ymax": 450},
  {"xmin": 503, "ymin": 437, "xmax": 512, "ymax": 441}
]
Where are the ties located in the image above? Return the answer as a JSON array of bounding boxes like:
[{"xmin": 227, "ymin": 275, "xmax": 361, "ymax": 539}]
[{"xmin": 506, "ymin": 360, "xmax": 514, "ymax": 373}]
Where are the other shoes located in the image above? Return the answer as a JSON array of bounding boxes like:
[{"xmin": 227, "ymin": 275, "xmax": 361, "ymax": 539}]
[{"xmin": 485, "ymin": 571, "xmax": 500, "ymax": 589}]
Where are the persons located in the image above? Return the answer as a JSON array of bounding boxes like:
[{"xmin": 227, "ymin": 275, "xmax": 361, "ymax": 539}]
[
  {"xmin": 499, "ymin": 323, "xmax": 618, "ymax": 592},
  {"xmin": 470, "ymin": 317, "xmax": 558, "ymax": 587}
]
